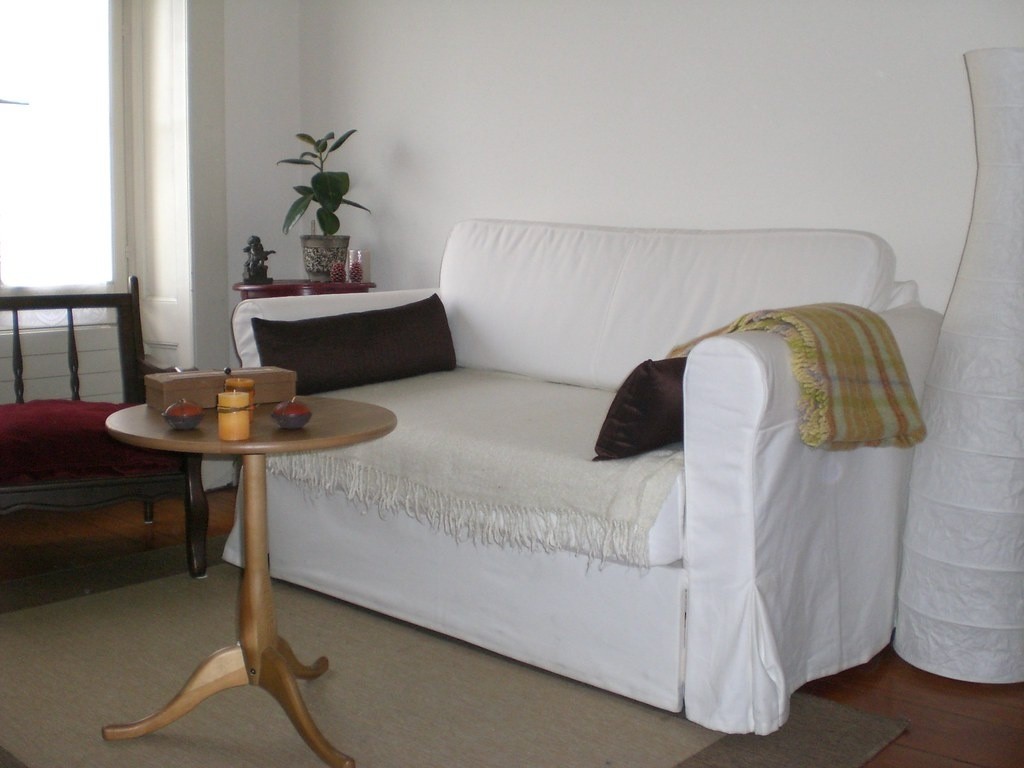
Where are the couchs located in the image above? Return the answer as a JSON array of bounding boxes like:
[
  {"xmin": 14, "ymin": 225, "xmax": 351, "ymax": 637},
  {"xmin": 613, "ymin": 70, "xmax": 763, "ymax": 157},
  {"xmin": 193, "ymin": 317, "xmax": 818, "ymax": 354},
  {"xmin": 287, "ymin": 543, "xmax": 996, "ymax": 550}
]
[{"xmin": 220, "ymin": 221, "xmax": 944, "ymax": 735}]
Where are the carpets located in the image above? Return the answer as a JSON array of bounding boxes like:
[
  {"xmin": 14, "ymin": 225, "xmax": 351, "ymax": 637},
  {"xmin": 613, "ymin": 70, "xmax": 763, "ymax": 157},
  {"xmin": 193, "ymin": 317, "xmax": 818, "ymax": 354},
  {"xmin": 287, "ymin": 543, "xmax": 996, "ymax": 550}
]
[{"xmin": 0, "ymin": 532, "xmax": 911, "ymax": 768}]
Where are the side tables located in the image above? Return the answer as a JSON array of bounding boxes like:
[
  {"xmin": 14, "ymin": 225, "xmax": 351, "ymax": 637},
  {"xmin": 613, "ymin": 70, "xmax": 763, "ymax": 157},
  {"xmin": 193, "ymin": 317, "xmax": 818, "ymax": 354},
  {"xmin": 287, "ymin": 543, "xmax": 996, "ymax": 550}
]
[{"xmin": 233, "ymin": 280, "xmax": 376, "ymax": 301}]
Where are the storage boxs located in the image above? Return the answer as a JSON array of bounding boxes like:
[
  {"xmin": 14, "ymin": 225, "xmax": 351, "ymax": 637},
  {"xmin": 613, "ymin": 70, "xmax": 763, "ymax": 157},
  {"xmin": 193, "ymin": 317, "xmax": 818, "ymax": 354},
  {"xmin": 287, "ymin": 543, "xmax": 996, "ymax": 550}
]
[{"xmin": 146, "ymin": 366, "xmax": 298, "ymax": 412}]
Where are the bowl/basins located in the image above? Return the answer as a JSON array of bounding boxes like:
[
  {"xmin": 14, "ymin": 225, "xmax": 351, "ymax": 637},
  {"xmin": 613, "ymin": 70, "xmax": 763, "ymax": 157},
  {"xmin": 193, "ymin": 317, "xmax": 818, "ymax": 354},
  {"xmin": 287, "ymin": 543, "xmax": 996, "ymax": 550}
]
[
  {"xmin": 161, "ymin": 411, "xmax": 207, "ymax": 432},
  {"xmin": 268, "ymin": 410, "xmax": 312, "ymax": 431}
]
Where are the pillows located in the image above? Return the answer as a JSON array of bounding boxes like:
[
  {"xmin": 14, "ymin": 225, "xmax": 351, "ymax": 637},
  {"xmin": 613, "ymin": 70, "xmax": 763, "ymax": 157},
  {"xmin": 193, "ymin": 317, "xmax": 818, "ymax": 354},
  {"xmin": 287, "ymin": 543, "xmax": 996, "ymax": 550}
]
[
  {"xmin": 592, "ymin": 356, "xmax": 687, "ymax": 461},
  {"xmin": 250, "ymin": 292, "xmax": 456, "ymax": 395}
]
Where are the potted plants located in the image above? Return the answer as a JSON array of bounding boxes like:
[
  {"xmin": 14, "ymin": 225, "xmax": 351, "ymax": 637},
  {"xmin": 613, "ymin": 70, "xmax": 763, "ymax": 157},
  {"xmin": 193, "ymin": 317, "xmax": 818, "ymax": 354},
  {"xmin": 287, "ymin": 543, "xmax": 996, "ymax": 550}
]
[{"xmin": 276, "ymin": 130, "xmax": 371, "ymax": 282}]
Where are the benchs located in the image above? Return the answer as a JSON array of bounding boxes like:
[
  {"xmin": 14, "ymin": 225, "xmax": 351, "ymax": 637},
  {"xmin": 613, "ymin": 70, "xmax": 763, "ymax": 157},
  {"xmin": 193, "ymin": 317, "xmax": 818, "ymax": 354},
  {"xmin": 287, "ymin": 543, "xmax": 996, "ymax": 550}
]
[{"xmin": 0, "ymin": 276, "xmax": 209, "ymax": 578}]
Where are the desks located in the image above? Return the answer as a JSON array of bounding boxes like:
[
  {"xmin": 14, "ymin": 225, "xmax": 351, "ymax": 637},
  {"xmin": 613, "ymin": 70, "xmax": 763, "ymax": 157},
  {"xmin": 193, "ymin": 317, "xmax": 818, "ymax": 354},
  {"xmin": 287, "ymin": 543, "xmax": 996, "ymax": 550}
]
[{"xmin": 102, "ymin": 396, "xmax": 397, "ymax": 768}]
